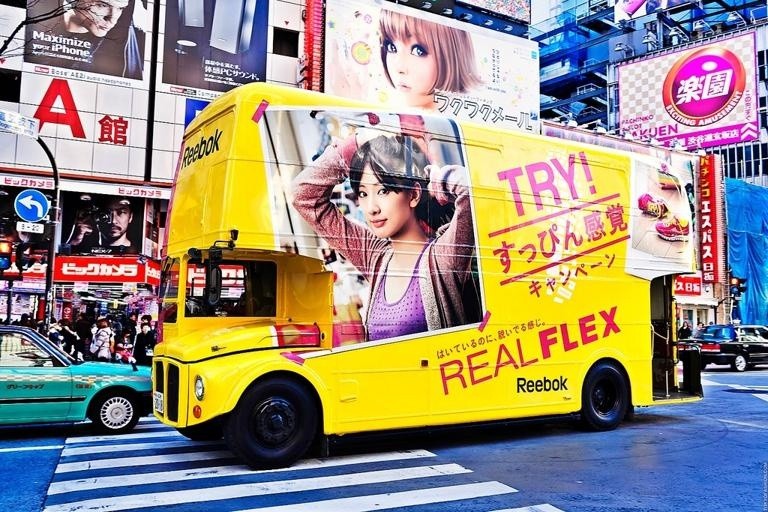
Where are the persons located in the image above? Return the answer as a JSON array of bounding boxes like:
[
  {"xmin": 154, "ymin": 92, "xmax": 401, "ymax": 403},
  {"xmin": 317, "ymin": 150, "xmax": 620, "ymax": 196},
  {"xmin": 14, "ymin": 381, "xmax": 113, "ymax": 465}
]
[
  {"xmin": 5, "ymin": 244, "xmax": 35, "ymax": 274},
  {"xmin": 56, "ymin": 195, "xmax": 140, "ymax": 256},
  {"xmin": 1, "ymin": 306, "xmax": 156, "ymax": 368},
  {"xmin": 25, "ymin": 0, "xmax": 143, "ymax": 78},
  {"xmin": 694, "ymin": 321, "xmax": 704, "ymax": 338},
  {"xmin": 677, "ymin": 321, "xmax": 691, "ymax": 340},
  {"xmin": 373, "ymin": 10, "xmax": 490, "ymax": 113},
  {"xmin": 285, "ymin": 114, "xmax": 485, "ymax": 343}
]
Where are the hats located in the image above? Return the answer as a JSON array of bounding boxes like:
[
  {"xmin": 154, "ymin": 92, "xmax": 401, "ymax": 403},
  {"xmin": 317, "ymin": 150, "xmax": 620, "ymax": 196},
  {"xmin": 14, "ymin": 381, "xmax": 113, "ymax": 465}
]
[
  {"xmin": 1, "ymin": 325, "xmax": 153, "ymax": 430},
  {"xmin": 677, "ymin": 325, "xmax": 768, "ymax": 372}
]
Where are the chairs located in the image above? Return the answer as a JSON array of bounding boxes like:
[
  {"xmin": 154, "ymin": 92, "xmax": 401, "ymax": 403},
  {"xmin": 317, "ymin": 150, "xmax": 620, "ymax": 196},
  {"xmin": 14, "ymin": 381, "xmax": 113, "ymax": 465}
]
[{"xmin": 638, "ymin": 193, "xmax": 690, "ymax": 242}]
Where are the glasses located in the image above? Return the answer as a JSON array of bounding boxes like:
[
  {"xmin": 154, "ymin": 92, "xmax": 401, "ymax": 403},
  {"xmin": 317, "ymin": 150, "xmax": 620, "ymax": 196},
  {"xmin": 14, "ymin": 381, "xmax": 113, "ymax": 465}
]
[{"xmin": 614, "ymin": 10, "xmax": 747, "ymax": 54}]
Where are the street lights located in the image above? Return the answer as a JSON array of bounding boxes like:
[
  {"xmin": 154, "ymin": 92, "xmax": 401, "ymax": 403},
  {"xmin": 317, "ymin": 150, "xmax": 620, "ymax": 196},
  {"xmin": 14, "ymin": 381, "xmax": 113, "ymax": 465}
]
[{"xmin": 146, "ymin": 350, "xmax": 153, "ymax": 356}]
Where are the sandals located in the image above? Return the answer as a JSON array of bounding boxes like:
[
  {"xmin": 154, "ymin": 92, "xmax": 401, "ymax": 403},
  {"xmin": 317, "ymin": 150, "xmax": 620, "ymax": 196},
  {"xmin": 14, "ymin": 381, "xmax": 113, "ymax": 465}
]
[{"xmin": 151, "ymin": 82, "xmax": 703, "ymax": 469}]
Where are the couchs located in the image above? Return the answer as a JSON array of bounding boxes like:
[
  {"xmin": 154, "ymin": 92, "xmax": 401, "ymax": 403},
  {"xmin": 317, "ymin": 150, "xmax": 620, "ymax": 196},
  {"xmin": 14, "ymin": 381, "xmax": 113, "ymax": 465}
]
[{"xmin": 730, "ymin": 277, "xmax": 747, "ymax": 296}]
